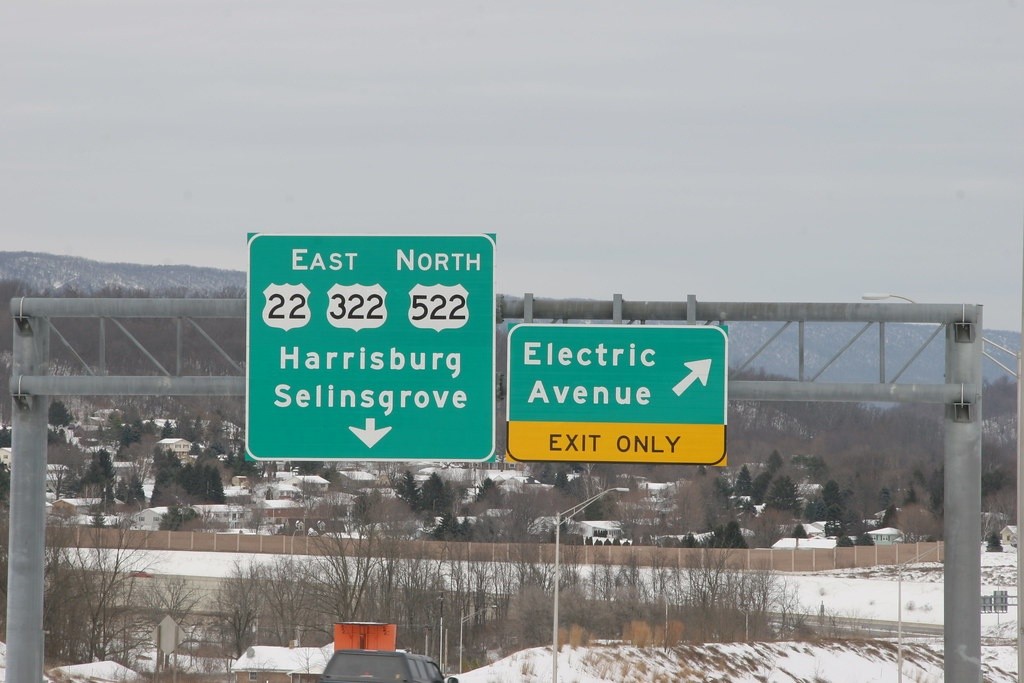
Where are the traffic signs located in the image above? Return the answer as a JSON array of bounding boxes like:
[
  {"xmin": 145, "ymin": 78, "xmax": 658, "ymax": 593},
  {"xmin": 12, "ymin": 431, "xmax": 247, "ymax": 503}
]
[
  {"xmin": 243, "ymin": 233, "xmax": 497, "ymax": 464},
  {"xmin": 505, "ymin": 324, "xmax": 730, "ymax": 466}
]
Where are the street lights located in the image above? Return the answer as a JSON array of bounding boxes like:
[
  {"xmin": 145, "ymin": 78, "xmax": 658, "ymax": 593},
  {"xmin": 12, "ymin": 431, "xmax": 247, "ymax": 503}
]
[
  {"xmin": 552, "ymin": 487, "xmax": 629, "ymax": 683},
  {"xmin": 861, "ymin": 293, "xmax": 1024, "ymax": 683},
  {"xmin": 459, "ymin": 605, "xmax": 497, "ymax": 675}
]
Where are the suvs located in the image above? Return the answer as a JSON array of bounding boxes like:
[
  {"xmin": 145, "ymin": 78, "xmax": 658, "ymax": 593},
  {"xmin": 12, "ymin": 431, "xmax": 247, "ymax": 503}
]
[{"xmin": 318, "ymin": 650, "xmax": 459, "ymax": 683}]
